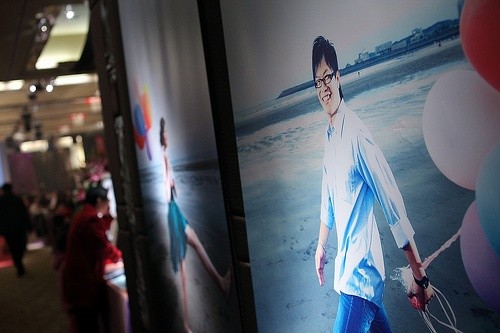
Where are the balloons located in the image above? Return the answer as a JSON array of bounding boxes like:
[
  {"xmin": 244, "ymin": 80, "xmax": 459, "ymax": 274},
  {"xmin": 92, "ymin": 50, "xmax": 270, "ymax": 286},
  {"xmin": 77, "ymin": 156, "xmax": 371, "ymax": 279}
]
[
  {"xmin": 130, "ymin": 85, "xmax": 155, "ymax": 163},
  {"xmin": 421, "ymin": 0, "xmax": 500, "ymax": 314}
]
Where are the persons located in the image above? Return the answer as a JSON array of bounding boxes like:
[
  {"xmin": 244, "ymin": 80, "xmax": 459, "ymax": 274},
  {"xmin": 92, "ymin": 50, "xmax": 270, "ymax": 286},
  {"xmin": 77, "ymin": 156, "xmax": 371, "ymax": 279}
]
[
  {"xmin": 311, "ymin": 35, "xmax": 434, "ymax": 333},
  {"xmin": 160, "ymin": 120, "xmax": 234, "ymax": 333},
  {"xmin": 0, "ymin": 180, "xmax": 123, "ymax": 333}
]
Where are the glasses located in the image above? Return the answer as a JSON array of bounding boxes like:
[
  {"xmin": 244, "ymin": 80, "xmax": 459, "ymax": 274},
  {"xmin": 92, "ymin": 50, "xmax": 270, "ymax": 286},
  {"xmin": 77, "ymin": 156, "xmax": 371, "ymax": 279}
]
[{"xmin": 314, "ymin": 72, "xmax": 337, "ymax": 88}]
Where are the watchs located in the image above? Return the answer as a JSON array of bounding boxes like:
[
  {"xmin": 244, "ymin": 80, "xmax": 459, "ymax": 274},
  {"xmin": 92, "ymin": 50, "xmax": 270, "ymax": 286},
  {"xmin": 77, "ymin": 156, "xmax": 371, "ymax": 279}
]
[{"xmin": 413, "ymin": 275, "xmax": 429, "ymax": 289}]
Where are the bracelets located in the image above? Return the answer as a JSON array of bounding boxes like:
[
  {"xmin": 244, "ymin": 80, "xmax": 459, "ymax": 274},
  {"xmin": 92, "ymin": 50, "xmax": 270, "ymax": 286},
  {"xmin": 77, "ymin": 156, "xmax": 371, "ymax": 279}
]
[{"xmin": 319, "ymin": 241, "xmax": 326, "ymax": 252}]
